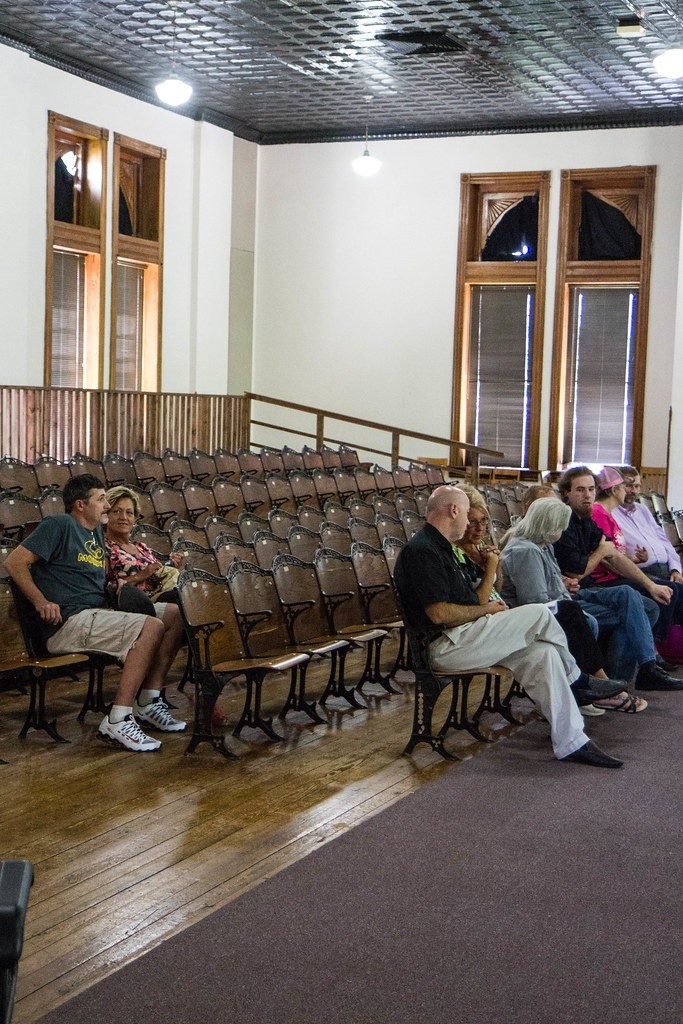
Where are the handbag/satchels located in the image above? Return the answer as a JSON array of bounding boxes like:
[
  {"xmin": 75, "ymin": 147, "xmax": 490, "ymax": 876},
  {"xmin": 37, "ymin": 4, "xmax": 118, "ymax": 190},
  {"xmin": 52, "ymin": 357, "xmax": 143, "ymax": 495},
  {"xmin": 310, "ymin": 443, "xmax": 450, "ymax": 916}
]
[
  {"xmin": 145, "ymin": 566, "xmax": 180, "ymax": 602},
  {"xmin": 100, "ymin": 586, "xmax": 156, "ymax": 617}
]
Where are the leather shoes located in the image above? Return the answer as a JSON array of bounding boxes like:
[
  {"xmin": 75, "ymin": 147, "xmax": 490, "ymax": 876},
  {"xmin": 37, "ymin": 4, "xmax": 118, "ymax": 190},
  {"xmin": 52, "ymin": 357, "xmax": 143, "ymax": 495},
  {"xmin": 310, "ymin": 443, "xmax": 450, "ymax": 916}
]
[
  {"xmin": 572, "ymin": 675, "xmax": 627, "ymax": 706},
  {"xmin": 635, "ymin": 666, "xmax": 683, "ymax": 691},
  {"xmin": 654, "ymin": 655, "xmax": 679, "ymax": 670},
  {"xmin": 563, "ymin": 740, "xmax": 624, "ymax": 768}
]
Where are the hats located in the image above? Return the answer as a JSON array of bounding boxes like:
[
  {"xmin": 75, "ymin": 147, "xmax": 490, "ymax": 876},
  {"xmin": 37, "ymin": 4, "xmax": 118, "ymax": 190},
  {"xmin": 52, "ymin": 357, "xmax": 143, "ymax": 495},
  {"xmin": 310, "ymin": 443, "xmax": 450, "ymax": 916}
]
[{"xmin": 596, "ymin": 467, "xmax": 625, "ymax": 490}]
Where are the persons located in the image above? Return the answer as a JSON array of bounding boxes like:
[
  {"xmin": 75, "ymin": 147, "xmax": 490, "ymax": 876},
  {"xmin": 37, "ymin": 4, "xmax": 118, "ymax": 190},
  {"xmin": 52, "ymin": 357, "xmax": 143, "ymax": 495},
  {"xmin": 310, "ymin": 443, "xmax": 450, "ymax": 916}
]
[
  {"xmin": 102, "ymin": 485, "xmax": 230, "ymax": 728},
  {"xmin": 393, "ymin": 465, "xmax": 683, "ymax": 769},
  {"xmin": 3, "ymin": 474, "xmax": 189, "ymax": 751}
]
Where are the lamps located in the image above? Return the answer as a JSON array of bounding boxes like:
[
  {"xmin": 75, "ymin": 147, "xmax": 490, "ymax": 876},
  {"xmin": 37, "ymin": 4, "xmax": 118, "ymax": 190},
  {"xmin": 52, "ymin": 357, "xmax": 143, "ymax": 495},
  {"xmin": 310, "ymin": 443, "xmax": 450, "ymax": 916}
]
[
  {"xmin": 155, "ymin": 1, "xmax": 193, "ymax": 106},
  {"xmin": 618, "ymin": 18, "xmax": 646, "ymax": 37}
]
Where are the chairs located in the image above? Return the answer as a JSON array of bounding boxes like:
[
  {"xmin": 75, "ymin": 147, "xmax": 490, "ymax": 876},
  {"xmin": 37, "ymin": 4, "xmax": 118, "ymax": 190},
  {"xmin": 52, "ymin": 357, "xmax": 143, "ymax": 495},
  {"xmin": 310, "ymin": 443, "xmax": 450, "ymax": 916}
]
[{"xmin": 1, "ymin": 442, "xmax": 683, "ymax": 763}]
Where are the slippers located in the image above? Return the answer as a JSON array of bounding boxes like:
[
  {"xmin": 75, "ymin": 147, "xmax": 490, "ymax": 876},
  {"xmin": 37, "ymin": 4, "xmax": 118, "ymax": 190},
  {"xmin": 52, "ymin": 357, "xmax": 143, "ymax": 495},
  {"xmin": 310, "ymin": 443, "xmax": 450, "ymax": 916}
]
[{"xmin": 593, "ymin": 694, "xmax": 648, "ymax": 713}]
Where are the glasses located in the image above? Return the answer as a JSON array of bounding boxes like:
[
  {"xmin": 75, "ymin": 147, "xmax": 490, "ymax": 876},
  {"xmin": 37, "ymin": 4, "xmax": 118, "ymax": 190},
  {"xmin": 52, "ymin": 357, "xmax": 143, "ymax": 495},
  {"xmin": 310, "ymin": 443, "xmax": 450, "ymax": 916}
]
[{"xmin": 467, "ymin": 517, "xmax": 488, "ymax": 529}]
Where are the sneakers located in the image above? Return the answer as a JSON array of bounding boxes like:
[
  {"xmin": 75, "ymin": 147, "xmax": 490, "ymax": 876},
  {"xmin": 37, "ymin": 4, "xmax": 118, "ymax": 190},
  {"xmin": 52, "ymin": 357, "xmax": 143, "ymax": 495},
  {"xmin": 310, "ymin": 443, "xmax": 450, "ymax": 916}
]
[
  {"xmin": 579, "ymin": 704, "xmax": 606, "ymax": 716},
  {"xmin": 96, "ymin": 715, "xmax": 163, "ymax": 752},
  {"xmin": 133, "ymin": 697, "xmax": 188, "ymax": 732}
]
